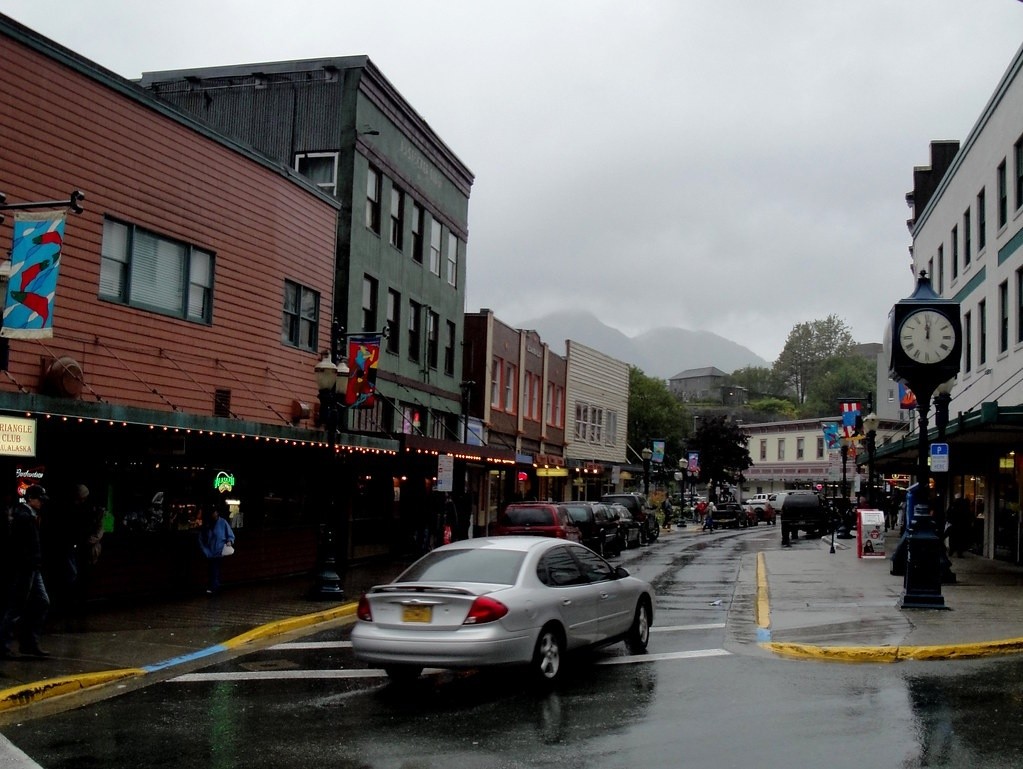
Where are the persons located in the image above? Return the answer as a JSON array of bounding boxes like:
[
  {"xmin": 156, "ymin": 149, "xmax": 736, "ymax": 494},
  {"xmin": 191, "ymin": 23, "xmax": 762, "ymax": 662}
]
[
  {"xmin": 198, "ymin": 505, "xmax": 236, "ymax": 594},
  {"xmin": 695, "ymin": 500, "xmax": 717, "ymax": 522},
  {"xmin": 0, "ymin": 486, "xmax": 50, "ymax": 656},
  {"xmin": 884, "ymin": 487, "xmax": 906, "ymax": 530},
  {"xmin": 766, "ymin": 504, "xmax": 776, "ymax": 525},
  {"xmin": 74, "ymin": 484, "xmax": 106, "ymax": 565},
  {"xmin": 703, "ymin": 509, "xmax": 713, "ymax": 532},
  {"xmin": 662, "ymin": 492, "xmax": 675, "ymax": 529}
]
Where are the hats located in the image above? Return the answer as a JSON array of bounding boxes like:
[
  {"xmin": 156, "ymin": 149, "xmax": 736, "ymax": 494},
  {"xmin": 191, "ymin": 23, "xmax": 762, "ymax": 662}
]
[
  {"xmin": 27, "ymin": 484, "xmax": 48, "ymax": 500},
  {"xmin": 870, "ymin": 531, "xmax": 878, "ymax": 534},
  {"xmin": 207, "ymin": 504, "xmax": 219, "ymax": 511}
]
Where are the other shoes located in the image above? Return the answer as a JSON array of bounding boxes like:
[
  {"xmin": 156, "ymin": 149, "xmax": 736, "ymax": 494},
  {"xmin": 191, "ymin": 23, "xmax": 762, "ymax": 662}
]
[
  {"xmin": 19, "ymin": 641, "xmax": 50, "ymax": 655},
  {"xmin": 0, "ymin": 649, "xmax": 24, "ymax": 661}
]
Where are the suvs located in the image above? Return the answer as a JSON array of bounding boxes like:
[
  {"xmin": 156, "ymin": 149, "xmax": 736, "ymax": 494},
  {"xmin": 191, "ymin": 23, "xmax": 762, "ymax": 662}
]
[
  {"xmin": 559, "ymin": 501, "xmax": 623, "ymax": 558},
  {"xmin": 601, "ymin": 502, "xmax": 643, "ymax": 548},
  {"xmin": 600, "ymin": 492, "xmax": 660, "ymax": 543},
  {"xmin": 781, "ymin": 491, "xmax": 832, "ymax": 536},
  {"xmin": 488, "ymin": 501, "xmax": 583, "ymax": 542}
]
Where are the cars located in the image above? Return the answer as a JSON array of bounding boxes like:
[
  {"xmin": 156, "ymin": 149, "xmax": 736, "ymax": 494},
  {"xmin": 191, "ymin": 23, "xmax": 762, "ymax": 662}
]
[
  {"xmin": 351, "ymin": 536, "xmax": 658, "ymax": 691},
  {"xmin": 742, "ymin": 504, "xmax": 758, "ymax": 527},
  {"xmin": 712, "ymin": 503, "xmax": 746, "ymax": 529},
  {"xmin": 752, "ymin": 502, "xmax": 776, "ymax": 525},
  {"xmin": 745, "ymin": 493, "xmax": 773, "ymax": 504}
]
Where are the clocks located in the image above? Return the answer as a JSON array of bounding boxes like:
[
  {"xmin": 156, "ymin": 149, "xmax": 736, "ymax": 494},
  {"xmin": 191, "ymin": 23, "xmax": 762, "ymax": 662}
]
[{"xmin": 899, "ymin": 307, "xmax": 955, "ymax": 365}]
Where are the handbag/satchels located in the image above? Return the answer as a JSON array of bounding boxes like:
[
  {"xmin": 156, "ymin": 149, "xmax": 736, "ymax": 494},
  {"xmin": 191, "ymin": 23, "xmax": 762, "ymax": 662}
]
[
  {"xmin": 703, "ymin": 516, "xmax": 713, "ymax": 526},
  {"xmin": 222, "ymin": 541, "xmax": 235, "ymax": 556},
  {"xmin": 666, "ymin": 508, "xmax": 673, "ymax": 515}
]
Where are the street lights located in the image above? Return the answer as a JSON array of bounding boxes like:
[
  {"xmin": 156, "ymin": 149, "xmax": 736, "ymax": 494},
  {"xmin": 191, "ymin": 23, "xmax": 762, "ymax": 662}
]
[
  {"xmin": 677, "ymin": 457, "xmax": 688, "ymax": 527},
  {"xmin": 687, "ymin": 470, "xmax": 698, "ymax": 508},
  {"xmin": 837, "ymin": 436, "xmax": 852, "ymax": 539},
  {"xmin": 932, "ymin": 376, "xmax": 956, "ymax": 584},
  {"xmin": 642, "ymin": 448, "xmax": 653, "ymax": 499},
  {"xmin": 863, "ymin": 412, "xmax": 879, "ymax": 509},
  {"xmin": 829, "ymin": 481, "xmax": 838, "ymax": 553},
  {"xmin": 308, "ymin": 358, "xmax": 349, "ymax": 602}
]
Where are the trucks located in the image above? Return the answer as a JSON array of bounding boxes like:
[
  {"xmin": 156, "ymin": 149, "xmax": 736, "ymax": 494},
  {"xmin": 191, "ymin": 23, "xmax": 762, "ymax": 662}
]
[{"xmin": 766, "ymin": 492, "xmax": 788, "ymax": 512}]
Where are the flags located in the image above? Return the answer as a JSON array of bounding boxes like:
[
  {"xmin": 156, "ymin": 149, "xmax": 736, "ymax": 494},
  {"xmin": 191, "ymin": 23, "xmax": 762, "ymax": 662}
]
[
  {"xmin": 0, "ymin": 210, "xmax": 67, "ymax": 339},
  {"xmin": 346, "ymin": 336, "xmax": 381, "ymax": 409}
]
